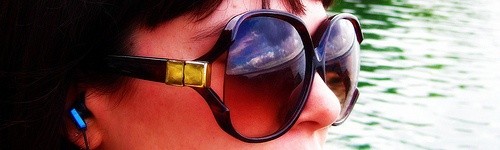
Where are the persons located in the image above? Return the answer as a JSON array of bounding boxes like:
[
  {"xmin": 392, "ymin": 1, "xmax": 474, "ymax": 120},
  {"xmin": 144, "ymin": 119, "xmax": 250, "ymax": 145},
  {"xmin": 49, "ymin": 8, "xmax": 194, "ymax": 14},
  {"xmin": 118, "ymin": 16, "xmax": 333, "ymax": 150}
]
[{"xmin": 0, "ymin": 0, "xmax": 364, "ymax": 150}]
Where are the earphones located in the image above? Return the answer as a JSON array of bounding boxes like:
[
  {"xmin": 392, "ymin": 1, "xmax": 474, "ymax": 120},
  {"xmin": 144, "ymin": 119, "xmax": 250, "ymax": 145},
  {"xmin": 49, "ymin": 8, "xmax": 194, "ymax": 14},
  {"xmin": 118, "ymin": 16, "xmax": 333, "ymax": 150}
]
[{"xmin": 70, "ymin": 92, "xmax": 94, "ymax": 130}]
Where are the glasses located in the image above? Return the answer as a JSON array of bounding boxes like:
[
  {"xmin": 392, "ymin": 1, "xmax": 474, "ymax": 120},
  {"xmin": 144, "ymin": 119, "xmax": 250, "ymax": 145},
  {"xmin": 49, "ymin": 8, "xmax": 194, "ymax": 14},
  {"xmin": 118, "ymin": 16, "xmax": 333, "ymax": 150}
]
[{"xmin": 107, "ymin": 10, "xmax": 364, "ymax": 143}]
